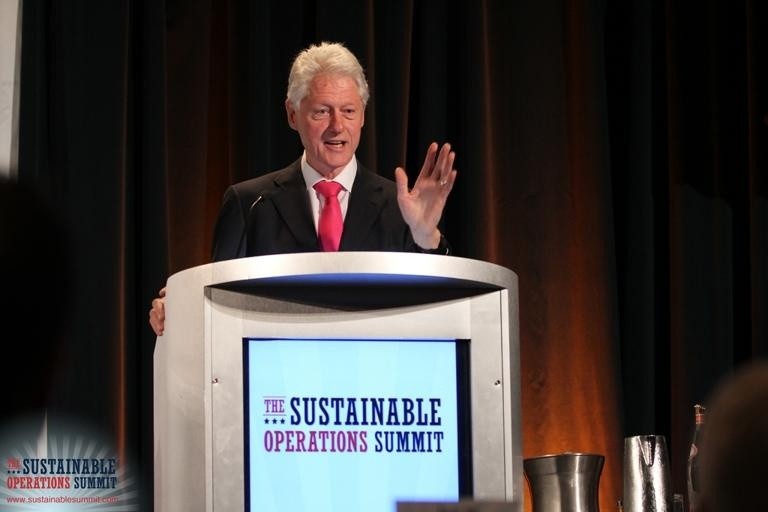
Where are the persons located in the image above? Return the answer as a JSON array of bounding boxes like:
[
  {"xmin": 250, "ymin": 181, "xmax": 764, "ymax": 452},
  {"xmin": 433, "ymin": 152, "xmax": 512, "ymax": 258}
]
[{"xmin": 145, "ymin": 40, "xmax": 462, "ymax": 337}]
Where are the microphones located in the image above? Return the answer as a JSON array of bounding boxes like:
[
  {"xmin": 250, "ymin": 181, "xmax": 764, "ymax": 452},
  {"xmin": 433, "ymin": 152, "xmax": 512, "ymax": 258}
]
[{"xmin": 234, "ymin": 190, "xmax": 271, "ymax": 258}]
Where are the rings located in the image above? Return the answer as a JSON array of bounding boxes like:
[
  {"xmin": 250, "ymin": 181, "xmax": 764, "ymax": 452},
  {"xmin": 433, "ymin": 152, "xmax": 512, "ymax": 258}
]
[{"xmin": 437, "ymin": 177, "xmax": 448, "ymax": 187}]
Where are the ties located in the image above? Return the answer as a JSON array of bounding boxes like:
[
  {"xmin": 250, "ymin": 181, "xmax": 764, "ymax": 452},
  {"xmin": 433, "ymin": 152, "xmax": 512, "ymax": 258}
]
[{"xmin": 313, "ymin": 180, "xmax": 343, "ymax": 252}]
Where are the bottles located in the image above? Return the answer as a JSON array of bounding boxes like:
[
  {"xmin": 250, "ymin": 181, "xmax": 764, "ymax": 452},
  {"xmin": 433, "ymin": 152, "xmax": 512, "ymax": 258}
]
[{"xmin": 687, "ymin": 404, "xmax": 724, "ymax": 511}]
